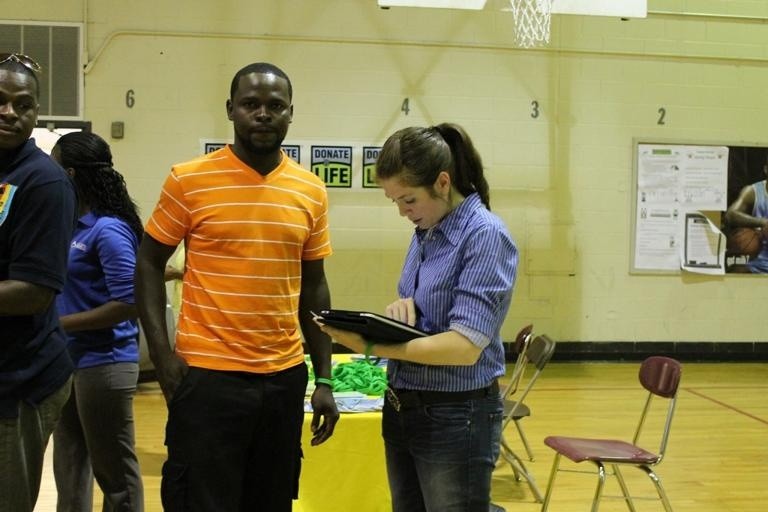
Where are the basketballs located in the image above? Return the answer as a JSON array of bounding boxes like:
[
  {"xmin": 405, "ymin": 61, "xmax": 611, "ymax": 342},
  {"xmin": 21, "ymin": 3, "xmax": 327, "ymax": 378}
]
[{"xmin": 729, "ymin": 227, "xmax": 761, "ymax": 255}]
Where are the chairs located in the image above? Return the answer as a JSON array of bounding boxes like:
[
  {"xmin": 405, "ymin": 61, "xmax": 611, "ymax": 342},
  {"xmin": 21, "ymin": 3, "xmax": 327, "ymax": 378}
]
[
  {"xmin": 494, "ymin": 331, "xmax": 564, "ymax": 507},
  {"xmin": 539, "ymin": 355, "xmax": 688, "ymax": 512},
  {"xmin": 495, "ymin": 323, "xmax": 535, "ymax": 459}
]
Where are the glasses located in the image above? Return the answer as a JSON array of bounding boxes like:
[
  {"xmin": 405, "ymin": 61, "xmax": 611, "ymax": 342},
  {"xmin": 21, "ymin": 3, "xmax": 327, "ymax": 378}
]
[{"xmin": 0, "ymin": 53, "xmax": 40, "ymax": 74}]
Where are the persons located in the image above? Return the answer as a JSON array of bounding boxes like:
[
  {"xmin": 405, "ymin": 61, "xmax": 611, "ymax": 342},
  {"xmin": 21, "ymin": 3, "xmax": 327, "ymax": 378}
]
[
  {"xmin": 161, "ymin": 240, "xmax": 186, "ymax": 331},
  {"xmin": 47, "ymin": 131, "xmax": 145, "ymax": 512},
  {"xmin": 134, "ymin": 62, "xmax": 341, "ymax": 512},
  {"xmin": 724, "ymin": 162, "xmax": 768, "ymax": 273},
  {"xmin": 1, "ymin": 52, "xmax": 76, "ymax": 512},
  {"xmin": 313, "ymin": 123, "xmax": 519, "ymax": 512}
]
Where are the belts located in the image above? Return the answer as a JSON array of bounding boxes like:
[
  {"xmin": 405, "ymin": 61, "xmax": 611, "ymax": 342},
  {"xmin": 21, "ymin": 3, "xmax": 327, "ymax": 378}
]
[{"xmin": 380, "ymin": 377, "xmax": 500, "ymax": 415}]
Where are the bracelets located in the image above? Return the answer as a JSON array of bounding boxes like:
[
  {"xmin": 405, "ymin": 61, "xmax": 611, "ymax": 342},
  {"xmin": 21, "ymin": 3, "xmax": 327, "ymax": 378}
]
[
  {"xmin": 364, "ymin": 341, "xmax": 381, "ymax": 367},
  {"xmin": 314, "ymin": 377, "xmax": 337, "ymax": 389}
]
